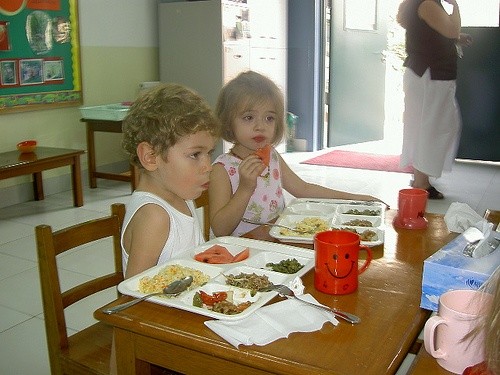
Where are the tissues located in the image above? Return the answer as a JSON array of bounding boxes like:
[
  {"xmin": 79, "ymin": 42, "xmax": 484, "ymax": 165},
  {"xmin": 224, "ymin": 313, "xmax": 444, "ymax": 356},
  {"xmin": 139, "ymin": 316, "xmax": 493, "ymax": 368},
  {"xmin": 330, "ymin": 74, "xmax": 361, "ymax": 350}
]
[{"xmin": 418, "ymin": 201, "xmax": 500, "ymax": 312}]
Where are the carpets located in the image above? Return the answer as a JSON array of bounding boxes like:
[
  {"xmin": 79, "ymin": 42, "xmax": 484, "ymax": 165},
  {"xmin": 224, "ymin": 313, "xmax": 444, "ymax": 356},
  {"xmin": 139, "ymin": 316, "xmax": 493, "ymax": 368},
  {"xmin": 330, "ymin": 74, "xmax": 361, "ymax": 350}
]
[{"xmin": 298, "ymin": 148, "xmax": 415, "ymax": 175}]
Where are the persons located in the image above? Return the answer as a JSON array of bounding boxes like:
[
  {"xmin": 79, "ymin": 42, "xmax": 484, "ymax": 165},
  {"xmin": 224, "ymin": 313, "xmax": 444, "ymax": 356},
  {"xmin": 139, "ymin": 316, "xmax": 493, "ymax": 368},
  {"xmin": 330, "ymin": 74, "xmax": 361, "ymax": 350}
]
[
  {"xmin": 120, "ymin": 85, "xmax": 224, "ymax": 280},
  {"xmin": 396, "ymin": 0, "xmax": 473, "ymax": 200},
  {"xmin": 209, "ymin": 72, "xmax": 389, "ymax": 238},
  {"xmin": 462, "ymin": 266, "xmax": 500, "ymax": 375}
]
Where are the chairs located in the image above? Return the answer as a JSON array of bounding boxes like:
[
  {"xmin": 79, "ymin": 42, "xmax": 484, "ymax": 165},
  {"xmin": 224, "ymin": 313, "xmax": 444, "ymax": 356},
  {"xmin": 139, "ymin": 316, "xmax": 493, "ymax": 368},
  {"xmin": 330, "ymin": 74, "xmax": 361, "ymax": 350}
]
[
  {"xmin": 34, "ymin": 202, "xmax": 154, "ymax": 375},
  {"xmin": 193, "ymin": 189, "xmax": 214, "ymax": 239}
]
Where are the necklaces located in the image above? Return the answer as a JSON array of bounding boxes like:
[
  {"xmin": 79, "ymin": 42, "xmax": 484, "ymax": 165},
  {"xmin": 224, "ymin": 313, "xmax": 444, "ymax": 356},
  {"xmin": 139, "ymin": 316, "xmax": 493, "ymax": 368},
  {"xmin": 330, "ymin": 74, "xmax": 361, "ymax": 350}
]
[{"xmin": 231, "ymin": 149, "xmax": 270, "ymax": 178}]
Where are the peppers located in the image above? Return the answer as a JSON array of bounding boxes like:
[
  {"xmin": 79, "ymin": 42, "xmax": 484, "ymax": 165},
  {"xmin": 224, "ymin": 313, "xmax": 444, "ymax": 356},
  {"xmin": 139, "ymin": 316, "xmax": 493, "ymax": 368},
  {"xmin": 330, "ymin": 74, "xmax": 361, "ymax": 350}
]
[{"xmin": 193, "ymin": 291, "xmax": 228, "ymax": 308}]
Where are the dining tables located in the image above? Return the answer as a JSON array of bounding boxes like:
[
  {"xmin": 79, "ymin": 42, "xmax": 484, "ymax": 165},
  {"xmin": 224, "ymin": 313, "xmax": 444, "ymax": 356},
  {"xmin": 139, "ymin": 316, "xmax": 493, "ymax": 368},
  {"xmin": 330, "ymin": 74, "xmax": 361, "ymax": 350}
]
[{"xmin": 93, "ymin": 206, "xmax": 464, "ymax": 375}]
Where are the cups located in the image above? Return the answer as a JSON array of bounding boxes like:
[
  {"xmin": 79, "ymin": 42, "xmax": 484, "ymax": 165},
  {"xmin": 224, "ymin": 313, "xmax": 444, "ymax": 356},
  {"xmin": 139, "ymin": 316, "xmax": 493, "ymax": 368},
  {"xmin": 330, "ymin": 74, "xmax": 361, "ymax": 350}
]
[
  {"xmin": 313, "ymin": 230, "xmax": 371, "ymax": 296},
  {"xmin": 392, "ymin": 189, "xmax": 429, "ymax": 230},
  {"xmin": 423, "ymin": 289, "xmax": 495, "ymax": 375}
]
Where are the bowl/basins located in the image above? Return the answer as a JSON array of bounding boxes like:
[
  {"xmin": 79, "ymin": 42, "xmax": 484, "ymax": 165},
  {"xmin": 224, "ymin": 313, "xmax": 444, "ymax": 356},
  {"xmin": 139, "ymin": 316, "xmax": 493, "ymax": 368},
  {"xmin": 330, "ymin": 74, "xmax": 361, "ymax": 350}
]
[{"xmin": 16, "ymin": 140, "xmax": 38, "ymax": 153}]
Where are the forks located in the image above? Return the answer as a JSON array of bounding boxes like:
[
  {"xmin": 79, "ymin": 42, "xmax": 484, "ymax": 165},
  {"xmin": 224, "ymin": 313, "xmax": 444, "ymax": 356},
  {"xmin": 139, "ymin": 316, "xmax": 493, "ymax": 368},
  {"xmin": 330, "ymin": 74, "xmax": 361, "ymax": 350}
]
[{"xmin": 258, "ymin": 285, "xmax": 361, "ymax": 324}]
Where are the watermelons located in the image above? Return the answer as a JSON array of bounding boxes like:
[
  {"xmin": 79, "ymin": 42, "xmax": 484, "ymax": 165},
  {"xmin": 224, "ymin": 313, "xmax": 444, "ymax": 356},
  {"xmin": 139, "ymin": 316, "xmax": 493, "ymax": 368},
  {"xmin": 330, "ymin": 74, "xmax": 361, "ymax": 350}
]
[
  {"xmin": 254, "ymin": 144, "xmax": 269, "ymax": 166},
  {"xmin": 195, "ymin": 245, "xmax": 249, "ymax": 264}
]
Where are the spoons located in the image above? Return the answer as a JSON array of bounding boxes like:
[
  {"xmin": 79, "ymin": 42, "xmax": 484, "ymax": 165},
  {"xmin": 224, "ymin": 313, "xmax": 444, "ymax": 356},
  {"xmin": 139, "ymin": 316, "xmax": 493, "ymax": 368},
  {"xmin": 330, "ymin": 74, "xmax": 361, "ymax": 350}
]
[
  {"xmin": 102, "ymin": 276, "xmax": 193, "ymax": 315},
  {"xmin": 240, "ymin": 217, "xmax": 317, "ymax": 233}
]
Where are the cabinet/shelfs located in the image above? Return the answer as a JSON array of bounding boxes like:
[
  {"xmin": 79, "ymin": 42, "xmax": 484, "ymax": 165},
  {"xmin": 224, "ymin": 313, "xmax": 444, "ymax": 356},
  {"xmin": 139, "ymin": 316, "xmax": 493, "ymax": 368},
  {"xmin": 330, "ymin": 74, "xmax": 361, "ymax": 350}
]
[{"xmin": 155, "ymin": 0, "xmax": 289, "ymax": 154}]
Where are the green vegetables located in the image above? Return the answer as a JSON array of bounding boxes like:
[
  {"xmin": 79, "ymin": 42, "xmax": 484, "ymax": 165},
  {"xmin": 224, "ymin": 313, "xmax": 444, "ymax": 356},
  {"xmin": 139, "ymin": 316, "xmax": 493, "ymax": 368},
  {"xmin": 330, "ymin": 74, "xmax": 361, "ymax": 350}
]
[{"xmin": 266, "ymin": 258, "xmax": 304, "ymax": 274}]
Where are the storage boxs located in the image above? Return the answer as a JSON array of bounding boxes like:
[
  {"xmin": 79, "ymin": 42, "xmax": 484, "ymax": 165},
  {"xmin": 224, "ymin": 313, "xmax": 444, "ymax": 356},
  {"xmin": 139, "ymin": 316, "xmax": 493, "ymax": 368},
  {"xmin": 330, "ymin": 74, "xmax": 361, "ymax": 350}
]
[{"xmin": 77, "ymin": 103, "xmax": 130, "ymax": 121}]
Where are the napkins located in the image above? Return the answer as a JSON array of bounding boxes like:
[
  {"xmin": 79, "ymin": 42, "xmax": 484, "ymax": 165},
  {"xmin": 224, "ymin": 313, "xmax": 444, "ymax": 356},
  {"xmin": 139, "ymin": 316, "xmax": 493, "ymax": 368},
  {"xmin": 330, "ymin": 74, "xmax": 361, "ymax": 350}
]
[{"xmin": 204, "ymin": 293, "xmax": 338, "ymax": 350}]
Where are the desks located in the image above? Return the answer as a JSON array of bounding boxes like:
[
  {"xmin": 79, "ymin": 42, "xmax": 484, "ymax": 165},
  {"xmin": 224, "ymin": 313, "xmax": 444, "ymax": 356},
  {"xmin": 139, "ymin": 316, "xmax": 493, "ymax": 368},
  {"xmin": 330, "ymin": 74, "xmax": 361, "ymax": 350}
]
[
  {"xmin": 79, "ymin": 117, "xmax": 140, "ymax": 195},
  {"xmin": 0, "ymin": 145, "xmax": 88, "ymax": 218}
]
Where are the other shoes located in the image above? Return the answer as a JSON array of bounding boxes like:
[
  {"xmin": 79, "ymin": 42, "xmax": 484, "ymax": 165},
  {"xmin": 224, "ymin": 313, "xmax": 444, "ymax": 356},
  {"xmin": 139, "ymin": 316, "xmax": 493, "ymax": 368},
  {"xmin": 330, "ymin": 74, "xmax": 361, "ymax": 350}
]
[{"xmin": 408, "ymin": 180, "xmax": 445, "ymax": 200}]
